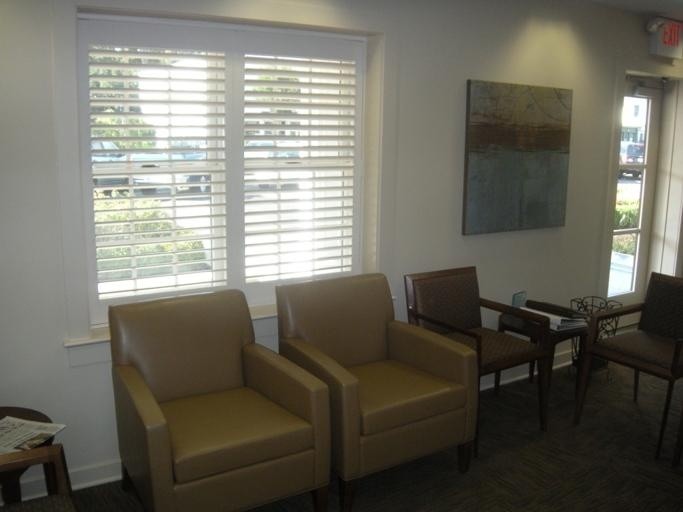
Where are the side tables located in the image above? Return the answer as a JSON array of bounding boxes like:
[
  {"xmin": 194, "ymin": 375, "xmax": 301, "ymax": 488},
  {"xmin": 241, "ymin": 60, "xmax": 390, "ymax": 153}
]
[
  {"xmin": 1, "ymin": 404, "xmax": 54, "ymax": 501},
  {"xmin": 495, "ymin": 312, "xmax": 600, "ymax": 434}
]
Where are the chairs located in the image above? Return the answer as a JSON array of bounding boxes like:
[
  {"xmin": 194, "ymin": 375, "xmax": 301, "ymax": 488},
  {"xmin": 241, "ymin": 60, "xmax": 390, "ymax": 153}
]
[
  {"xmin": 0, "ymin": 443, "xmax": 77, "ymax": 512},
  {"xmin": 402, "ymin": 265, "xmax": 545, "ymax": 463},
  {"xmin": 573, "ymin": 272, "xmax": 682, "ymax": 461},
  {"xmin": 275, "ymin": 273, "xmax": 480, "ymax": 512},
  {"xmin": 109, "ymin": 287, "xmax": 332, "ymax": 512}
]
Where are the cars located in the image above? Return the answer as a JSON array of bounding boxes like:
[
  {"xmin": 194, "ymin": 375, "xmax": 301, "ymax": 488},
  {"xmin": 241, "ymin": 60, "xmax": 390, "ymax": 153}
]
[
  {"xmin": 618, "ymin": 142, "xmax": 645, "ymax": 179},
  {"xmin": 90, "ymin": 138, "xmax": 306, "ymax": 196}
]
